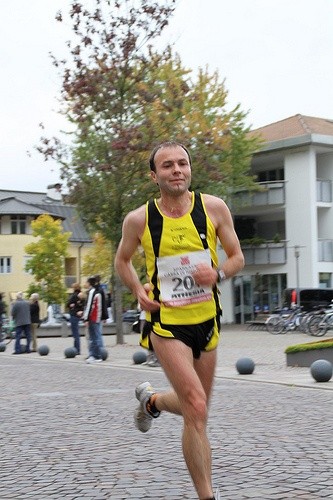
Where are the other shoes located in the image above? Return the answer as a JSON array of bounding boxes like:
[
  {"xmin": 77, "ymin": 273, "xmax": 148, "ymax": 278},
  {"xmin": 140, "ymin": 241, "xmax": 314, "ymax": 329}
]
[
  {"xmin": 95, "ymin": 358, "xmax": 103, "ymax": 363},
  {"xmin": 142, "ymin": 358, "xmax": 153, "ymax": 365},
  {"xmin": 77, "ymin": 353, "xmax": 80, "ymax": 355},
  {"xmin": 31, "ymin": 350, "xmax": 36, "ymax": 352},
  {"xmin": 83, "ymin": 355, "xmax": 96, "ymax": 364},
  {"xmin": 13, "ymin": 352, "xmax": 22, "ymax": 354},
  {"xmin": 148, "ymin": 358, "xmax": 160, "ymax": 367}
]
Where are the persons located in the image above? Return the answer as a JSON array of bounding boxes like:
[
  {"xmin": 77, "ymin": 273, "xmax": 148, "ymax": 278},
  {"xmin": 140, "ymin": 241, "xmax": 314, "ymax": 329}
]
[
  {"xmin": 0, "ymin": 275, "xmax": 161, "ymax": 366},
  {"xmin": 114, "ymin": 142, "xmax": 245, "ymax": 500}
]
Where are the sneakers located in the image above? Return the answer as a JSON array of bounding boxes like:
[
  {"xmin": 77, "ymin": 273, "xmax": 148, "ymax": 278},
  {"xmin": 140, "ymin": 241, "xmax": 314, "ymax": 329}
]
[{"xmin": 133, "ymin": 380, "xmax": 157, "ymax": 433}]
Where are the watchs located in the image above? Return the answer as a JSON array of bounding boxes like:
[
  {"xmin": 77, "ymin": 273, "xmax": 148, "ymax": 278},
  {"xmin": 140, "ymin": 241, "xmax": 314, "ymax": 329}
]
[{"xmin": 215, "ymin": 267, "xmax": 226, "ymax": 283}]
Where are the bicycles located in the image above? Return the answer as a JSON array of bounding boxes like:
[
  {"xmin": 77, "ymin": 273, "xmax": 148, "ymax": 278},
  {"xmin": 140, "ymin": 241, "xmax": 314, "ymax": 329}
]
[{"xmin": 265, "ymin": 301, "xmax": 333, "ymax": 338}]
